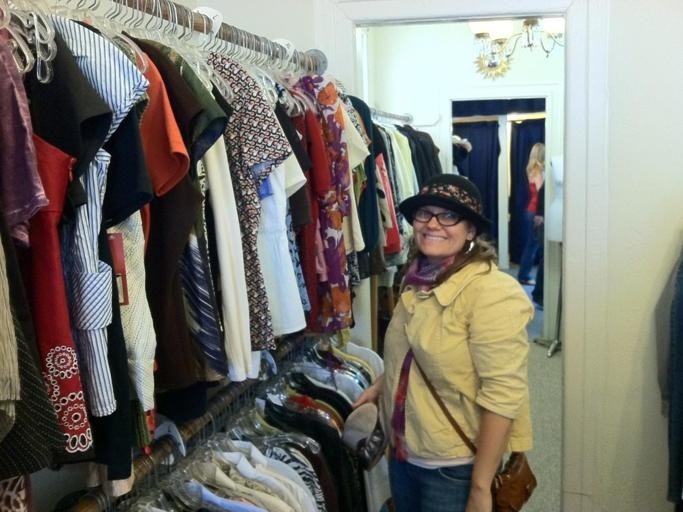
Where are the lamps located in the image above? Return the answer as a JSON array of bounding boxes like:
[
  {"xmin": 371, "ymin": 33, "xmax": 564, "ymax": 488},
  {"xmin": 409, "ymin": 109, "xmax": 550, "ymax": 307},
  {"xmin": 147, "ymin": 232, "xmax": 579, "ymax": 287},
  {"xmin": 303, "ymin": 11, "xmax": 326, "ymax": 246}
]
[{"xmin": 473, "ymin": 16, "xmax": 564, "ymax": 80}]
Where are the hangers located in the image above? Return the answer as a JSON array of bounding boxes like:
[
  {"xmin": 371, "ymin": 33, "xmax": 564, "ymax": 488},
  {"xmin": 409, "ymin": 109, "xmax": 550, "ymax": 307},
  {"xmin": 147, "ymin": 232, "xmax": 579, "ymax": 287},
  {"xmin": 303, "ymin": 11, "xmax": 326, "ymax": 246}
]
[
  {"xmin": 86, "ymin": 335, "xmax": 346, "ymax": 512},
  {"xmin": 0, "ymin": 1, "xmax": 318, "ymax": 116}
]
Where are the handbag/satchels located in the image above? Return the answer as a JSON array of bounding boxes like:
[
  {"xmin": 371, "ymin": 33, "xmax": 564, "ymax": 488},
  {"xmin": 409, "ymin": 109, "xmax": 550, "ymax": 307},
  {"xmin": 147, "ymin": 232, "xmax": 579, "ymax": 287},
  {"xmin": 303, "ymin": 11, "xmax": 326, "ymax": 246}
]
[{"xmin": 490, "ymin": 451, "xmax": 539, "ymax": 512}]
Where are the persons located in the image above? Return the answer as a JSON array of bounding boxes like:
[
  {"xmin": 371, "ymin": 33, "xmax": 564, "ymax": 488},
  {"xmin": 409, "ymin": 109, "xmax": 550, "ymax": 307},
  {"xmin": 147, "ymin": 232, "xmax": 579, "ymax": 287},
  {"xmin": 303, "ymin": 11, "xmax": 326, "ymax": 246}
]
[
  {"xmin": 516, "ymin": 142, "xmax": 545, "ymax": 285},
  {"xmin": 531, "ymin": 181, "xmax": 544, "ymax": 312},
  {"xmin": 350, "ymin": 175, "xmax": 534, "ymax": 512}
]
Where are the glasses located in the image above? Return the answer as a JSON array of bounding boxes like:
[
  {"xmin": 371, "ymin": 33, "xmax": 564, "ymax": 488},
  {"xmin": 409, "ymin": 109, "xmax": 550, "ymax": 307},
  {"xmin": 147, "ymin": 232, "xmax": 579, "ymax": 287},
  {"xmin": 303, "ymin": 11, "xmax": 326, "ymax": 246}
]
[{"xmin": 412, "ymin": 208, "xmax": 466, "ymax": 227}]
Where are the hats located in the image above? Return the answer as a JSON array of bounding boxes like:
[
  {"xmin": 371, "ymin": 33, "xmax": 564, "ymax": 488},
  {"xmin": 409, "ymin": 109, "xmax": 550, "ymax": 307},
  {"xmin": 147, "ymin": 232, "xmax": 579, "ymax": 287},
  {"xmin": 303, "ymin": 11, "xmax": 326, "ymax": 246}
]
[{"xmin": 399, "ymin": 172, "xmax": 489, "ymax": 226}]
